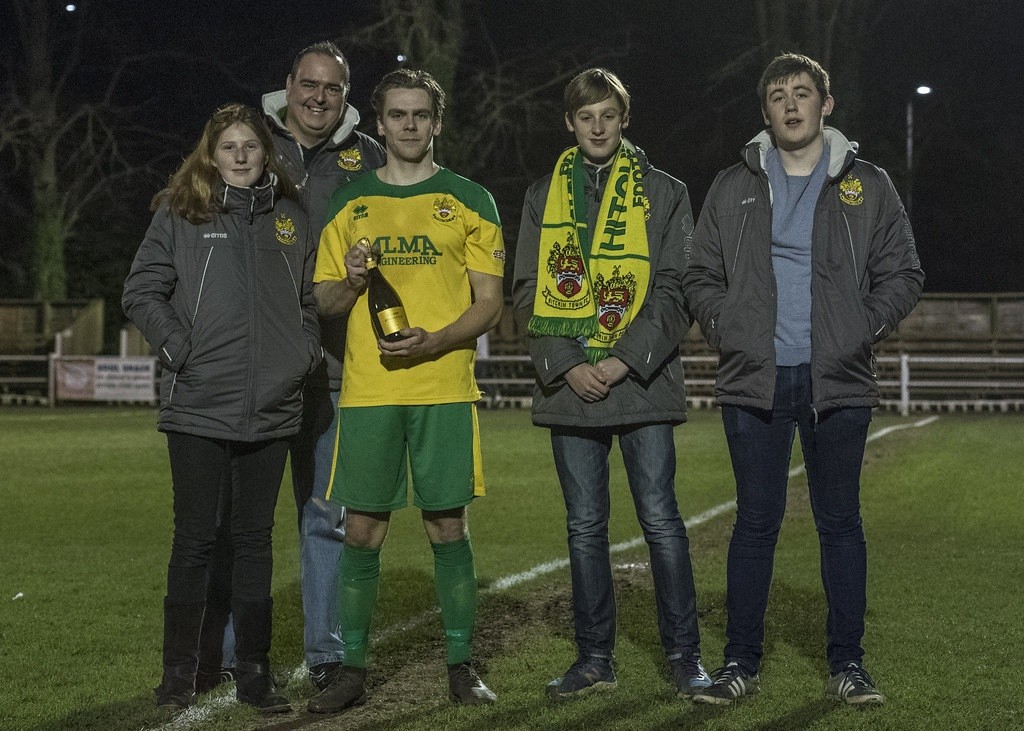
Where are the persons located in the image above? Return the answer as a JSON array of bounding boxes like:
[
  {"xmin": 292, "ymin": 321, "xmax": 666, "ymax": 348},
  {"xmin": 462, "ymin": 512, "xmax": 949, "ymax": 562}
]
[
  {"xmin": 118, "ymin": 100, "xmax": 329, "ymax": 717},
  {"xmin": 681, "ymin": 51, "xmax": 928, "ymax": 706},
  {"xmin": 194, "ymin": 39, "xmax": 390, "ymax": 698},
  {"xmin": 512, "ymin": 65, "xmax": 716, "ymax": 701},
  {"xmin": 303, "ymin": 67, "xmax": 506, "ymax": 715}
]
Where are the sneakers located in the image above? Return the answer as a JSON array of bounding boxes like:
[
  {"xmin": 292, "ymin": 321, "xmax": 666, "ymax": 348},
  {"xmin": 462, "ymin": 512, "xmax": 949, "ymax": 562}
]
[
  {"xmin": 660, "ymin": 648, "xmax": 711, "ymax": 693},
  {"xmin": 306, "ymin": 660, "xmax": 342, "ymax": 691},
  {"xmin": 545, "ymin": 654, "xmax": 616, "ymax": 698},
  {"xmin": 694, "ymin": 660, "xmax": 761, "ymax": 704},
  {"xmin": 824, "ymin": 663, "xmax": 884, "ymax": 706},
  {"xmin": 447, "ymin": 662, "xmax": 499, "ymax": 707},
  {"xmin": 194, "ymin": 666, "xmax": 236, "ymax": 692},
  {"xmin": 307, "ymin": 664, "xmax": 369, "ymax": 714}
]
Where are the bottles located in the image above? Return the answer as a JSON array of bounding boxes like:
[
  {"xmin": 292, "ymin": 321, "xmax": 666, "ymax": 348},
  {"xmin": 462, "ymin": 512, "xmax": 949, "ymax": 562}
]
[{"xmin": 358, "ymin": 237, "xmax": 412, "ymax": 342}]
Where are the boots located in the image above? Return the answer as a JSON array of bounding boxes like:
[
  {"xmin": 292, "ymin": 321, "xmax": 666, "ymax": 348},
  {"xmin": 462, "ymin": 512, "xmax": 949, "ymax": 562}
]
[
  {"xmin": 155, "ymin": 597, "xmax": 207, "ymax": 712},
  {"xmin": 228, "ymin": 594, "xmax": 292, "ymax": 712}
]
